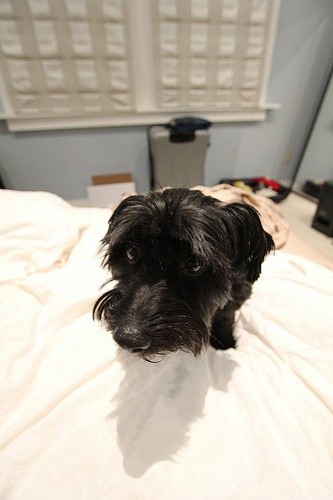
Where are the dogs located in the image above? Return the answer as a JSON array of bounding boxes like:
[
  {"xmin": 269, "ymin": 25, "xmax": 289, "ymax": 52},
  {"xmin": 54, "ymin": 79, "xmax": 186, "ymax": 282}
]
[{"xmin": 90, "ymin": 187, "xmax": 276, "ymax": 363}]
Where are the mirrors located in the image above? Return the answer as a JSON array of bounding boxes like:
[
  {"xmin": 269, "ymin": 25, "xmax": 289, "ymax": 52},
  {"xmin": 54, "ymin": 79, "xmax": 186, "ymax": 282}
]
[{"xmin": 289, "ymin": 65, "xmax": 333, "ymax": 205}]
